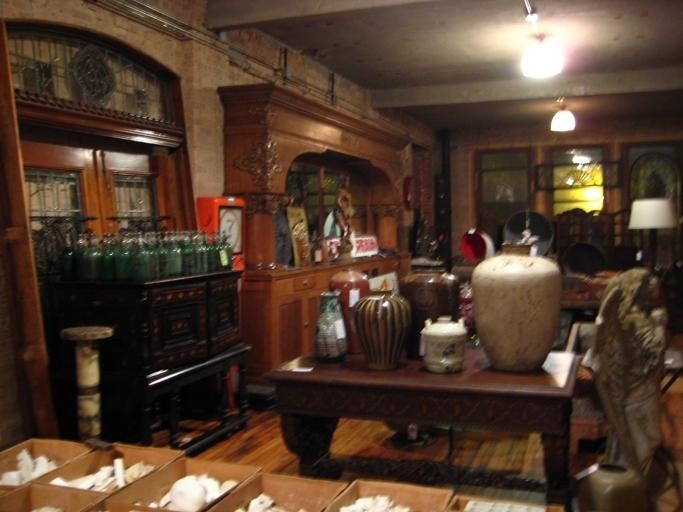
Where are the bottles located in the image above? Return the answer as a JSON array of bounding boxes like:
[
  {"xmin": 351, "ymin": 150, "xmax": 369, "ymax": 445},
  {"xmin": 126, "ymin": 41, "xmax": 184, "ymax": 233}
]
[
  {"xmin": 70, "ymin": 230, "xmax": 234, "ymax": 276},
  {"xmin": 314, "ymin": 243, "xmax": 564, "ymax": 374}
]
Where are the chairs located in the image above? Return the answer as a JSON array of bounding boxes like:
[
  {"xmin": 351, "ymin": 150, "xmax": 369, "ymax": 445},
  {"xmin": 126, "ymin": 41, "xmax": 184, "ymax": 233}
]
[{"xmin": 506, "ymin": 210, "xmax": 613, "ymax": 274}]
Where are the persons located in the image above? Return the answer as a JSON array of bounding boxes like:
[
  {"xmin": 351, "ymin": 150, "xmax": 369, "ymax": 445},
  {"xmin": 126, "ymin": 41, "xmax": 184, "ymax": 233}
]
[{"xmin": 335, "ymin": 174, "xmax": 354, "ymax": 246}]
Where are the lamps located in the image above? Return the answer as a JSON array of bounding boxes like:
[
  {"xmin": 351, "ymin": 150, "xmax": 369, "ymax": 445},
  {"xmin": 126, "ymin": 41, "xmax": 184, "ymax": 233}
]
[
  {"xmin": 550, "ymin": 99, "xmax": 576, "ymax": 132},
  {"xmin": 627, "ymin": 196, "xmax": 678, "ymax": 266}
]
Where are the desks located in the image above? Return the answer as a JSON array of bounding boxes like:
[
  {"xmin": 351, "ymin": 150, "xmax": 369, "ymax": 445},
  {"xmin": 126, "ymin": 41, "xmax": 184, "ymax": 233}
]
[{"xmin": 262, "ymin": 336, "xmax": 587, "ymax": 512}]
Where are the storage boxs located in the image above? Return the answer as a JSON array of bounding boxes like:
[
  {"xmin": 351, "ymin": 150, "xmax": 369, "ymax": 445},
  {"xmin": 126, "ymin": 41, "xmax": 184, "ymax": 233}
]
[{"xmin": 1, "ymin": 438, "xmax": 563, "ymax": 512}]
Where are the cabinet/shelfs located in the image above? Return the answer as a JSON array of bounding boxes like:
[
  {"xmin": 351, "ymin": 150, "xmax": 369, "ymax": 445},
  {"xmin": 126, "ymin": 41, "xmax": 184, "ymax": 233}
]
[
  {"xmin": 52, "ymin": 268, "xmax": 255, "ymax": 455},
  {"xmin": 240, "ymin": 250, "xmax": 414, "ymax": 391}
]
